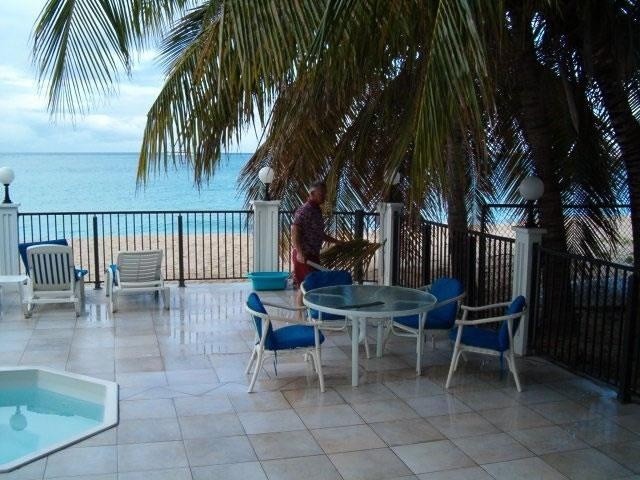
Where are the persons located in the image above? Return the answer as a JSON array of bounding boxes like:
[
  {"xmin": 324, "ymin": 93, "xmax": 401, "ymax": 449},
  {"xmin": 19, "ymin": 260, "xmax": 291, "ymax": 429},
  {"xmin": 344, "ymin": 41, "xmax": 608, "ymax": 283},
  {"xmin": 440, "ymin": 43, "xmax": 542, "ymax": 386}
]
[{"xmin": 289, "ymin": 183, "xmax": 346, "ymax": 322}]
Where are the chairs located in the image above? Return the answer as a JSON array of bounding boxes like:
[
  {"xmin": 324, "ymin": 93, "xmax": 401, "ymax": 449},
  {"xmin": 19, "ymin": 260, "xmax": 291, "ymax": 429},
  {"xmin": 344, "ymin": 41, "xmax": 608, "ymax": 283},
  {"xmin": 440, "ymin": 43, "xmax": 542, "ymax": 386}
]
[
  {"xmin": 26, "ymin": 244, "xmax": 171, "ymax": 319},
  {"xmin": 245, "ymin": 269, "xmax": 528, "ymax": 395}
]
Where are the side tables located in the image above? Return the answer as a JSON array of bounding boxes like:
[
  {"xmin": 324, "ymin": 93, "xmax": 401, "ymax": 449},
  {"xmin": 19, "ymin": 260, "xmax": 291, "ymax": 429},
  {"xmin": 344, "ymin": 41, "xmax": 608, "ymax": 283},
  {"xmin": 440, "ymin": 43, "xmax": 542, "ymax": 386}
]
[{"xmin": 0, "ymin": 275, "xmax": 29, "ymax": 319}]
